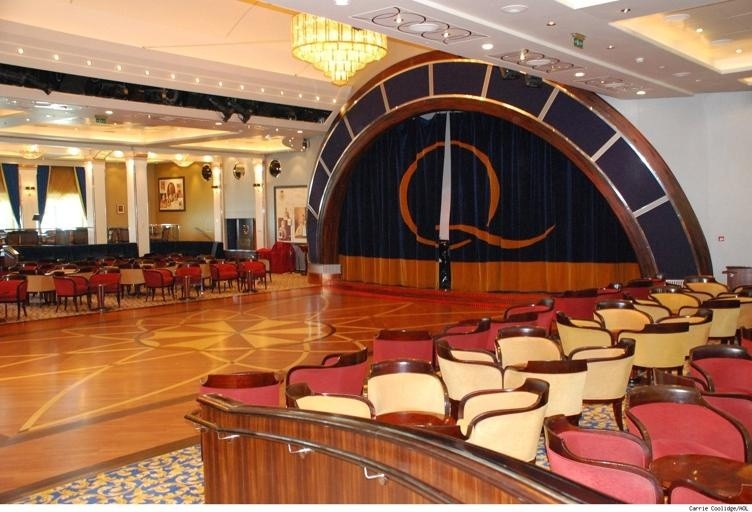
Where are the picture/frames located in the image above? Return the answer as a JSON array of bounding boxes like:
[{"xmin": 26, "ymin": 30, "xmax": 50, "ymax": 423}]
[
  {"xmin": 116, "ymin": 203, "xmax": 125, "ymax": 215},
  {"xmin": 274, "ymin": 184, "xmax": 309, "ymax": 244},
  {"xmin": 157, "ymin": 176, "xmax": 186, "ymax": 212}
]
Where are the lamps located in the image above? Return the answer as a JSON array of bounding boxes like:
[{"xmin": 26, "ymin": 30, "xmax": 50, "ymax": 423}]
[{"xmin": 290, "ymin": 12, "xmax": 388, "ymax": 88}]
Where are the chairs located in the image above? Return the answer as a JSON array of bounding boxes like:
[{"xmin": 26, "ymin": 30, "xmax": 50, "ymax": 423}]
[{"xmin": 1, "ymin": 255, "xmax": 271, "ymax": 319}]
